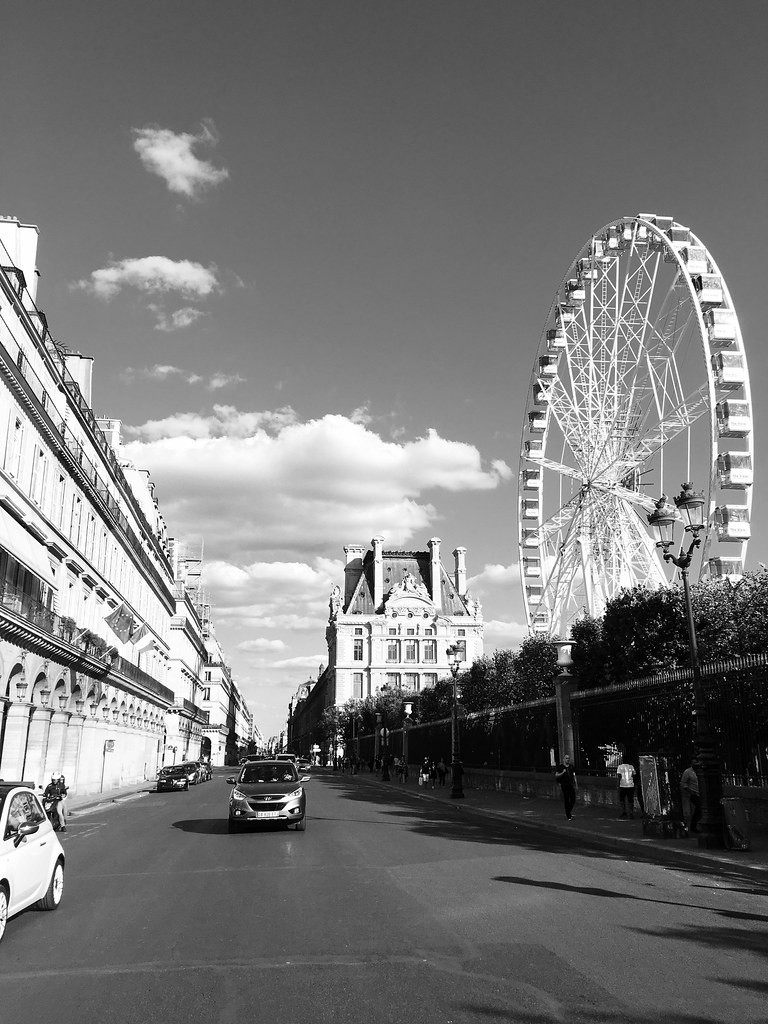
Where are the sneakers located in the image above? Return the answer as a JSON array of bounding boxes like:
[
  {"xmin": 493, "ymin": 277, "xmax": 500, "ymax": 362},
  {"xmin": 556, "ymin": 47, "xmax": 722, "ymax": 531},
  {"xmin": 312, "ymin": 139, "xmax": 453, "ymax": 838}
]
[
  {"xmin": 567, "ymin": 816, "xmax": 575, "ymax": 822},
  {"xmin": 61, "ymin": 826, "xmax": 66, "ymax": 832},
  {"xmin": 628, "ymin": 814, "xmax": 633, "ymax": 819},
  {"xmin": 619, "ymin": 813, "xmax": 627, "ymax": 819}
]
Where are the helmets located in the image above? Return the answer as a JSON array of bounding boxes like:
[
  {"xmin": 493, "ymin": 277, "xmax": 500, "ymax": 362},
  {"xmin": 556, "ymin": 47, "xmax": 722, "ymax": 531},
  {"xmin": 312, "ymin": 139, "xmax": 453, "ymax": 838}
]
[
  {"xmin": 60, "ymin": 775, "xmax": 65, "ymax": 781},
  {"xmin": 51, "ymin": 772, "xmax": 61, "ymax": 782}
]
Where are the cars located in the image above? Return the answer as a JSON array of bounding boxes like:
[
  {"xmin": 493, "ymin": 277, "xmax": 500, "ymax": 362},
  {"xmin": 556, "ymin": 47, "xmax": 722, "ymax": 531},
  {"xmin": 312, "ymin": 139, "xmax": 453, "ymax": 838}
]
[
  {"xmin": 225, "ymin": 760, "xmax": 311, "ymax": 834},
  {"xmin": 296, "ymin": 758, "xmax": 311, "ymax": 773},
  {"xmin": 276, "ymin": 754, "xmax": 297, "ymax": 771},
  {"xmin": 157, "ymin": 765, "xmax": 190, "ymax": 792},
  {"xmin": 0, "ymin": 780, "xmax": 66, "ymax": 941},
  {"xmin": 182, "ymin": 761, "xmax": 212, "ymax": 786}
]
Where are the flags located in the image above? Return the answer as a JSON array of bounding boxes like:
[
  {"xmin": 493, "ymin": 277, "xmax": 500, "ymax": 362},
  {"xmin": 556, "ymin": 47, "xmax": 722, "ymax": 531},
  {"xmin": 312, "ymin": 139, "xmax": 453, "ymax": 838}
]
[
  {"xmin": 102, "ymin": 601, "xmax": 136, "ymax": 645},
  {"xmin": 130, "ymin": 622, "xmax": 154, "ymax": 651}
]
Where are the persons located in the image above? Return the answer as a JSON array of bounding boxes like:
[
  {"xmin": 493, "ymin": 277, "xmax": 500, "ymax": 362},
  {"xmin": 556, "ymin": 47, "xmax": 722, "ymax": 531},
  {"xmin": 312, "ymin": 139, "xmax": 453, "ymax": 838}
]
[
  {"xmin": 420, "ymin": 756, "xmax": 464, "ymax": 790},
  {"xmin": 199, "ymin": 754, "xmax": 209, "ymax": 762},
  {"xmin": 332, "ymin": 755, "xmax": 365, "ymax": 775},
  {"xmin": 662, "ymin": 758, "xmax": 704, "ymax": 835},
  {"xmin": 555, "ymin": 754, "xmax": 578, "ymax": 820},
  {"xmin": 319, "ymin": 755, "xmax": 329, "ymax": 767},
  {"xmin": 368, "ymin": 753, "xmax": 408, "ymax": 784},
  {"xmin": 617, "ymin": 755, "xmax": 636, "ymax": 820},
  {"xmin": 45, "ymin": 773, "xmax": 68, "ymax": 832}
]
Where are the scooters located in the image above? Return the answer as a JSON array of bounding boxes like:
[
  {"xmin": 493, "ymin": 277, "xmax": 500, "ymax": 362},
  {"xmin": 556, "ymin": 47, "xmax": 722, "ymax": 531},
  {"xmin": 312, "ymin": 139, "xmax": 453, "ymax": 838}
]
[{"xmin": 38, "ymin": 784, "xmax": 69, "ymax": 831}]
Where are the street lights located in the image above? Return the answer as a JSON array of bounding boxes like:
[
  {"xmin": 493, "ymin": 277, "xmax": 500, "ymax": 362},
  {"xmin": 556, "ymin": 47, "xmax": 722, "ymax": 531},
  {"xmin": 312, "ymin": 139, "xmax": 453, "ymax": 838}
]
[
  {"xmin": 648, "ymin": 486, "xmax": 730, "ymax": 851},
  {"xmin": 445, "ymin": 643, "xmax": 466, "ymax": 798}
]
